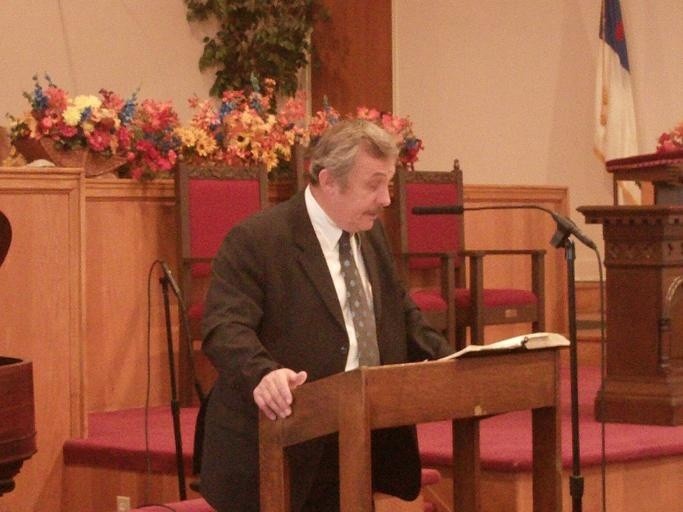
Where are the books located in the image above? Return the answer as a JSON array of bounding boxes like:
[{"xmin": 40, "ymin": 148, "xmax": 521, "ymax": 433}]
[{"xmin": 439, "ymin": 331, "xmax": 571, "ymax": 362}]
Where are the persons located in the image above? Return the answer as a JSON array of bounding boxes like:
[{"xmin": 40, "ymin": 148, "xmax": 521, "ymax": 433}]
[{"xmin": 189, "ymin": 119, "xmax": 453, "ymax": 512}]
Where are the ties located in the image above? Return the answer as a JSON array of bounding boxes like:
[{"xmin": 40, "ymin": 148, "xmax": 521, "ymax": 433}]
[{"xmin": 339, "ymin": 231, "xmax": 381, "ymax": 367}]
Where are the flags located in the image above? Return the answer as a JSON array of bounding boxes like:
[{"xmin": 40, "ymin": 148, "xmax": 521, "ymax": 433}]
[{"xmin": 592, "ymin": 0, "xmax": 642, "ymax": 204}]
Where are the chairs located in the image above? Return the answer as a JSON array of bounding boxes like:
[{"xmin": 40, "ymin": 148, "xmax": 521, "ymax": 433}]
[
  {"xmin": 174, "ymin": 142, "xmax": 271, "ymax": 419},
  {"xmin": 390, "ymin": 160, "xmax": 548, "ymax": 372}
]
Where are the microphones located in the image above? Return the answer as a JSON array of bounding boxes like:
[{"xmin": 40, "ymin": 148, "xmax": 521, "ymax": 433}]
[
  {"xmin": 411, "ymin": 204, "xmax": 593, "ymax": 249},
  {"xmin": 161, "ymin": 261, "xmax": 207, "ymax": 406}
]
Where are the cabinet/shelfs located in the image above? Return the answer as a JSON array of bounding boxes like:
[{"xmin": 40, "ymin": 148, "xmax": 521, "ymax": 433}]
[{"xmin": 575, "ymin": 150, "xmax": 683, "ymax": 427}]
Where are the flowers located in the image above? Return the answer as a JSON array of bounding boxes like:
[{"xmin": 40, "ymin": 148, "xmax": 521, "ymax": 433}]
[{"xmin": 7, "ymin": 76, "xmax": 425, "ymax": 180}]
